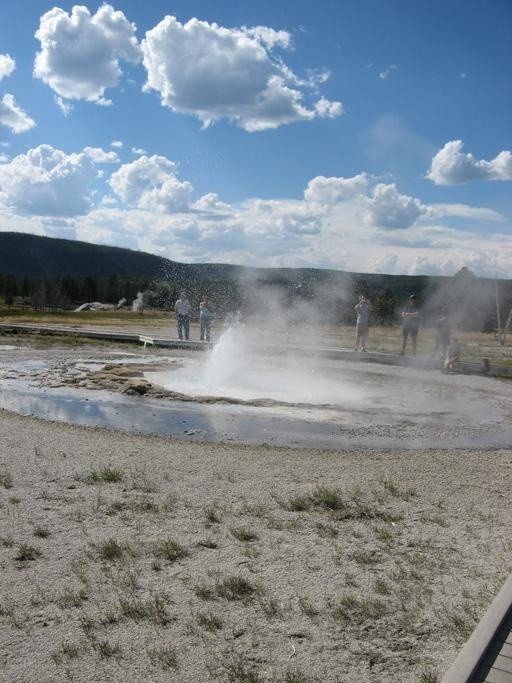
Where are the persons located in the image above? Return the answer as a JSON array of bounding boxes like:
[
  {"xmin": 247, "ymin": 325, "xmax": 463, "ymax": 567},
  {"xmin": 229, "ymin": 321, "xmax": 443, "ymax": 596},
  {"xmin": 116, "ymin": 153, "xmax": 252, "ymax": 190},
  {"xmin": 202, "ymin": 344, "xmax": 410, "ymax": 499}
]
[
  {"xmin": 353, "ymin": 294, "xmax": 375, "ymax": 353},
  {"xmin": 397, "ymin": 293, "xmax": 421, "ymax": 358},
  {"xmin": 198, "ymin": 294, "xmax": 215, "ymax": 341},
  {"xmin": 440, "ymin": 335, "xmax": 461, "ymax": 374},
  {"xmin": 428, "ymin": 306, "xmax": 452, "ymax": 360},
  {"xmin": 174, "ymin": 290, "xmax": 194, "ymax": 341}
]
